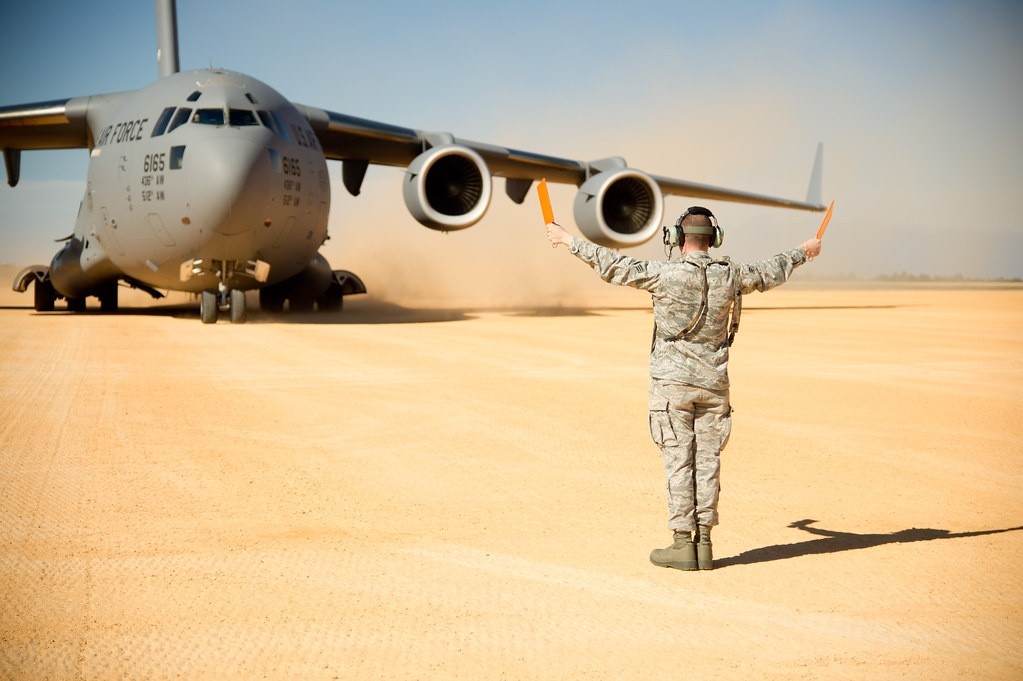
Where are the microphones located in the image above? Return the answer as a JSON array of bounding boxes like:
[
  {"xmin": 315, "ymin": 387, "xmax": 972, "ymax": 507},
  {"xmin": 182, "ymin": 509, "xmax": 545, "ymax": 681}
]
[{"xmin": 662, "ymin": 226, "xmax": 669, "ymax": 244}]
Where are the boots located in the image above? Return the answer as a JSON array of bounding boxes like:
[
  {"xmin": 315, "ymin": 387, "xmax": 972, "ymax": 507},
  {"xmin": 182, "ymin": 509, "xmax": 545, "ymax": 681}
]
[
  {"xmin": 650, "ymin": 530, "xmax": 697, "ymax": 570},
  {"xmin": 694, "ymin": 535, "xmax": 714, "ymax": 570}
]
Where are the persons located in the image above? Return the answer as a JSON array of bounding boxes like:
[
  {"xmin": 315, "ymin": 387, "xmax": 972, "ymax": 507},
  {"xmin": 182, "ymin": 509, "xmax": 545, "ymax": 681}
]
[{"xmin": 545, "ymin": 206, "xmax": 822, "ymax": 571}]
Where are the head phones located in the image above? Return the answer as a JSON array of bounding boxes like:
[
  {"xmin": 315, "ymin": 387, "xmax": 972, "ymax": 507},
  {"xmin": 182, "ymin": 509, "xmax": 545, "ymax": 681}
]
[{"xmin": 667, "ymin": 206, "xmax": 724, "ymax": 247}]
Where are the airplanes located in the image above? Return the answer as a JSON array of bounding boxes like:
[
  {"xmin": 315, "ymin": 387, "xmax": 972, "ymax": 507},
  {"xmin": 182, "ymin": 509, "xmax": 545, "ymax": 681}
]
[{"xmin": 0, "ymin": 0, "xmax": 828, "ymax": 327}]
